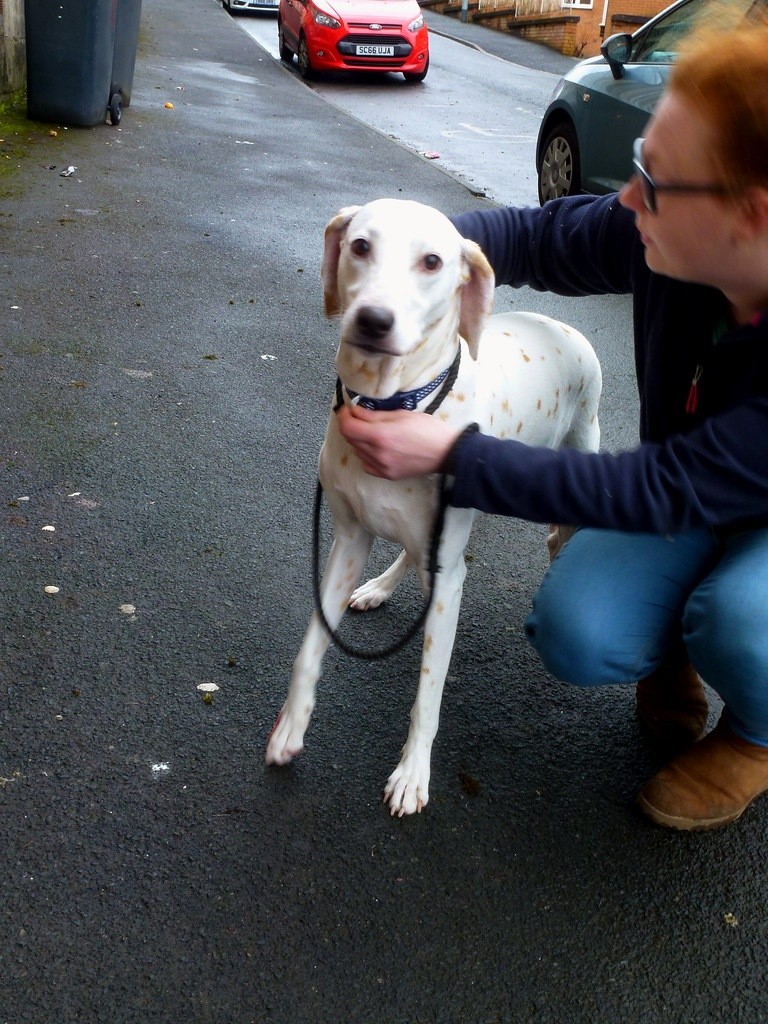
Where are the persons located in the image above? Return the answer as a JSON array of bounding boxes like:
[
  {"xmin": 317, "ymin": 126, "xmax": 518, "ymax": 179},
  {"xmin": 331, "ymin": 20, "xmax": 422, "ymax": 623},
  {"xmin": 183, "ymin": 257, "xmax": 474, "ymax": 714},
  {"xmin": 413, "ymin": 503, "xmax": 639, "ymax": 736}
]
[{"xmin": 336, "ymin": 0, "xmax": 768, "ymax": 830}]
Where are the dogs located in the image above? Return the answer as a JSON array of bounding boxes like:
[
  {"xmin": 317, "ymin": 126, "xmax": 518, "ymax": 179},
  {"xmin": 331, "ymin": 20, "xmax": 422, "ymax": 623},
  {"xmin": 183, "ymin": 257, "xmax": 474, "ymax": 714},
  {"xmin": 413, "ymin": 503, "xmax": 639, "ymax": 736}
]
[{"xmin": 266, "ymin": 197, "xmax": 604, "ymax": 818}]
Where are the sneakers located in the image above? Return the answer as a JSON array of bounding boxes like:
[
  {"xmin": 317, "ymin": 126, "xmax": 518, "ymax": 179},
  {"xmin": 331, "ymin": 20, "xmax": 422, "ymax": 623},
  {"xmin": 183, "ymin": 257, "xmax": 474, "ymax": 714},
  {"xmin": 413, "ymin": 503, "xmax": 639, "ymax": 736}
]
[
  {"xmin": 636, "ymin": 718, "xmax": 768, "ymax": 831},
  {"xmin": 636, "ymin": 665, "xmax": 709, "ymax": 741}
]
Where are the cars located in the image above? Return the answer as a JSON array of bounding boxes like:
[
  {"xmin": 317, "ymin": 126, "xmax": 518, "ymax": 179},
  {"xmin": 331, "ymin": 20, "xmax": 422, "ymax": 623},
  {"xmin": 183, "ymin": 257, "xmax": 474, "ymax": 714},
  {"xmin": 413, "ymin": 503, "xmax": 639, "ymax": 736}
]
[
  {"xmin": 274, "ymin": 0, "xmax": 432, "ymax": 84},
  {"xmin": 218, "ymin": 0, "xmax": 281, "ymax": 14},
  {"xmin": 535, "ymin": 0, "xmax": 768, "ymax": 206}
]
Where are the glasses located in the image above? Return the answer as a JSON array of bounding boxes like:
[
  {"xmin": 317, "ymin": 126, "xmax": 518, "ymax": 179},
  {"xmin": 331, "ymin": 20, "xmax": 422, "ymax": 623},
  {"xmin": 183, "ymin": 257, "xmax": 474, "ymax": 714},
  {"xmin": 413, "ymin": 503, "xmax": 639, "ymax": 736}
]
[{"xmin": 632, "ymin": 137, "xmax": 728, "ymax": 218}]
